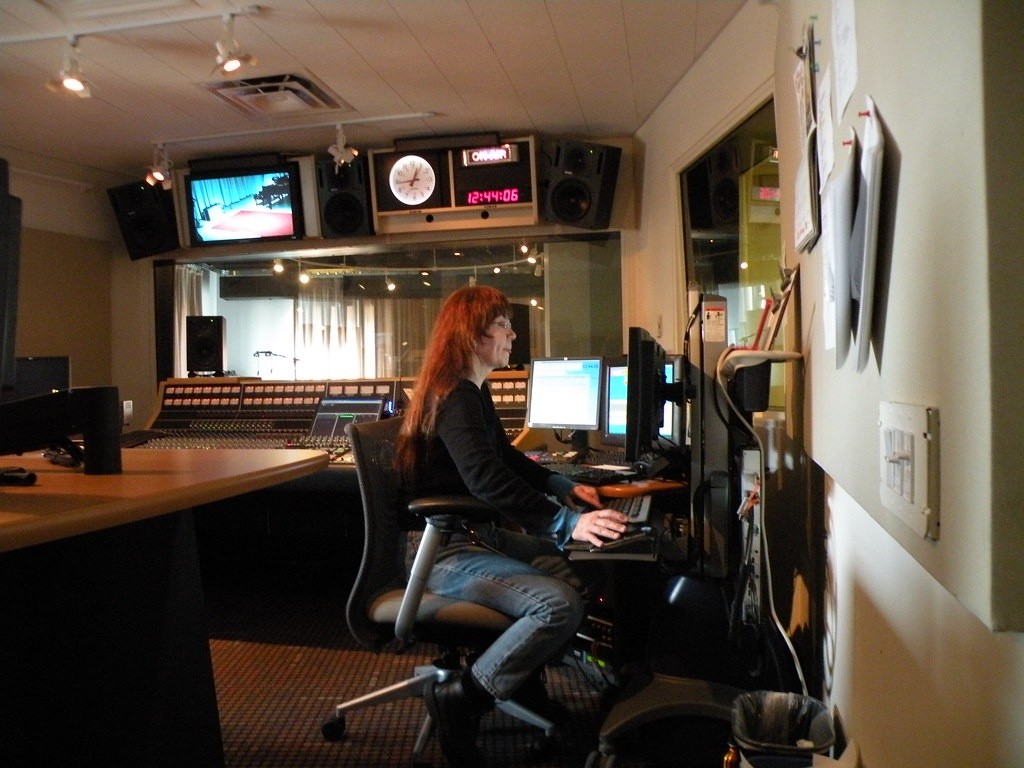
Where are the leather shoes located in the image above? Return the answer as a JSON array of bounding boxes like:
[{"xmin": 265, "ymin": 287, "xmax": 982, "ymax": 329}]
[{"xmin": 422, "ymin": 675, "xmax": 480, "ymax": 768}]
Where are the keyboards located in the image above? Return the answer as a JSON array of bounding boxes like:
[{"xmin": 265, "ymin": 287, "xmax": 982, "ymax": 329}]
[
  {"xmin": 542, "ymin": 463, "xmax": 626, "ymax": 487},
  {"xmin": 590, "ymin": 494, "xmax": 654, "ymax": 525}
]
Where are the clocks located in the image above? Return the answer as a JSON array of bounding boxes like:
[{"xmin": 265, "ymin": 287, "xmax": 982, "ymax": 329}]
[{"xmin": 388, "ymin": 154, "xmax": 436, "ymax": 206}]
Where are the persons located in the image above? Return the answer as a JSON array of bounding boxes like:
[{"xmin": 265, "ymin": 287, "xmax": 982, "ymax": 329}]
[{"xmin": 392, "ymin": 285, "xmax": 630, "ymax": 768}]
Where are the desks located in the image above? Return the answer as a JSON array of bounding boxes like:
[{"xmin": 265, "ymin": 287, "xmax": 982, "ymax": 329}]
[{"xmin": 0, "ymin": 447, "xmax": 331, "ymax": 768}]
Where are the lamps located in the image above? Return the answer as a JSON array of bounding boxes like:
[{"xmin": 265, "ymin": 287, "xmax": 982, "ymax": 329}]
[
  {"xmin": 521, "ymin": 239, "xmax": 543, "ymax": 277},
  {"xmin": 208, "ymin": 13, "xmax": 256, "ymax": 77},
  {"xmin": 469, "ymin": 266, "xmax": 477, "ymax": 287},
  {"xmin": 273, "ymin": 258, "xmax": 283, "ymax": 273},
  {"xmin": 299, "ymin": 262, "xmax": 310, "ymax": 283},
  {"xmin": 327, "ymin": 124, "xmax": 357, "ymax": 174},
  {"xmin": 44, "ymin": 33, "xmax": 101, "ymax": 99},
  {"xmin": 146, "ymin": 142, "xmax": 175, "ymax": 190},
  {"xmin": 384, "ymin": 272, "xmax": 395, "ymax": 291}
]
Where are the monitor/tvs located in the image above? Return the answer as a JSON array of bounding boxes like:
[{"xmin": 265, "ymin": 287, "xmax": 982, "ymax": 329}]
[
  {"xmin": 13, "ymin": 356, "xmax": 71, "ymax": 399},
  {"xmin": 527, "ymin": 324, "xmax": 685, "ymax": 461},
  {"xmin": 184, "ymin": 161, "xmax": 306, "ymax": 248}
]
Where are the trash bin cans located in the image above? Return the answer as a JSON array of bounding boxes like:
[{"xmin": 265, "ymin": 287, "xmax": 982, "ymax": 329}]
[{"xmin": 732, "ymin": 691, "xmax": 836, "ymax": 768}]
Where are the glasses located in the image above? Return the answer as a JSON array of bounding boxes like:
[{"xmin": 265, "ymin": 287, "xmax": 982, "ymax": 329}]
[{"xmin": 490, "ymin": 321, "xmax": 512, "ymax": 331}]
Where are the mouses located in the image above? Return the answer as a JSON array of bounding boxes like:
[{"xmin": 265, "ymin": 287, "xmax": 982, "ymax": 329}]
[{"xmin": 593, "ymin": 524, "xmax": 640, "ymax": 543}]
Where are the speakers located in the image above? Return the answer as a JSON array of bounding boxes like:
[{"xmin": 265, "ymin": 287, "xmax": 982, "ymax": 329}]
[
  {"xmin": 186, "ymin": 316, "xmax": 227, "ymax": 373},
  {"xmin": 686, "ymin": 134, "xmax": 740, "ymax": 234},
  {"xmin": 546, "ymin": 138, "xmax": 622, "ymax": 230},
  {"xmin": 106, "ymin": 178, "xmax": 180, "ymax": 261},
  {"xmin": 316, "ymin": 151, "xmax": 376, "ymax": 240}
]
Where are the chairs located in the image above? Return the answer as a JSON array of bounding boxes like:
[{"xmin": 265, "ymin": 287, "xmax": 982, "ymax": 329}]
[{"xmin": 322, "ymin": 415, "xmax": 555, "ymax": 768}]
